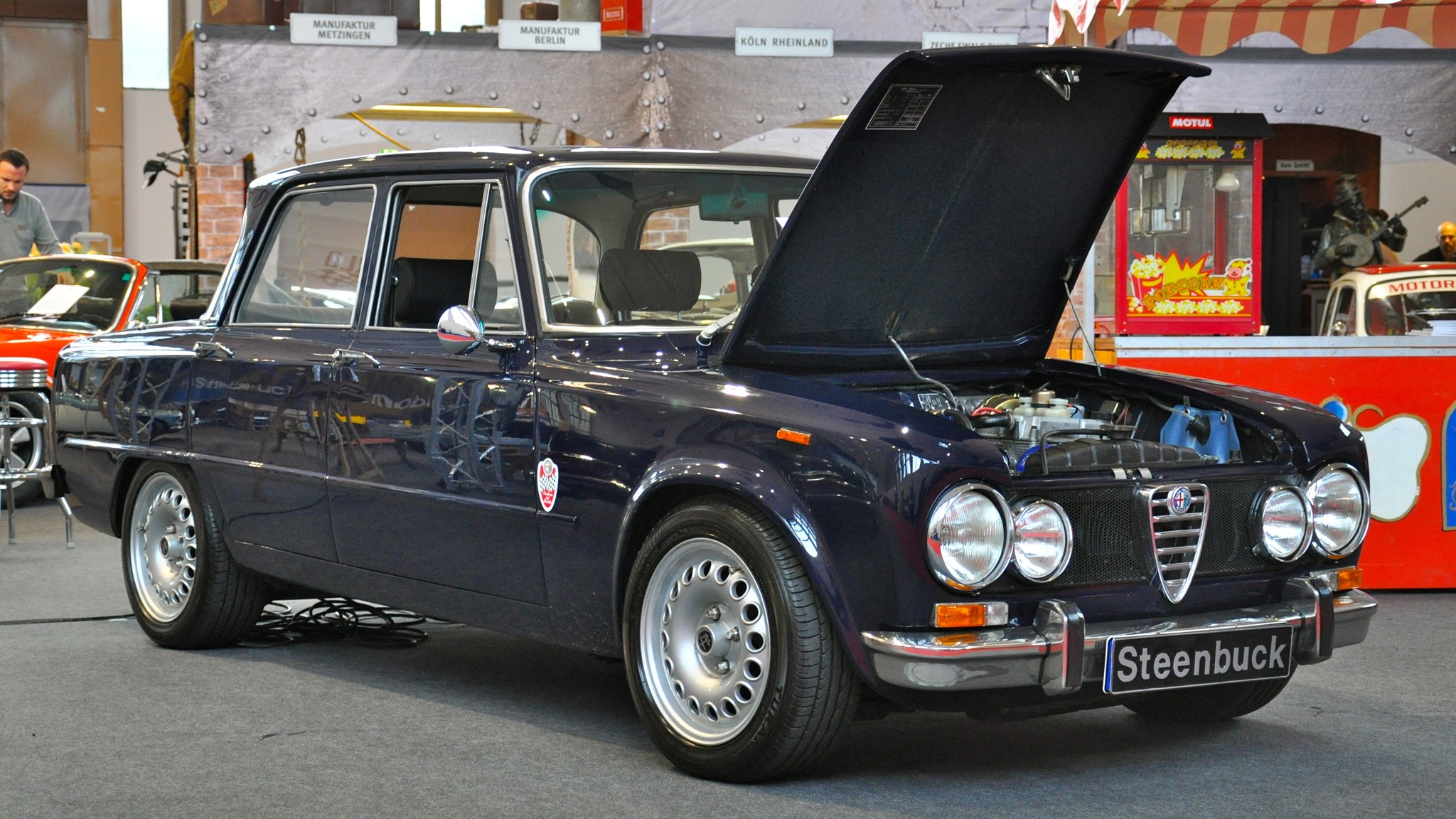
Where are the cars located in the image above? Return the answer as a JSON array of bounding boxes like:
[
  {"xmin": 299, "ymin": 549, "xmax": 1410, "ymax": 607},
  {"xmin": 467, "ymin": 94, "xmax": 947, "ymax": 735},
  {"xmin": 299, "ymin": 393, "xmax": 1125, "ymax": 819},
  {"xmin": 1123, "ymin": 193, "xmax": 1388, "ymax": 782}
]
[{"xmin": 44, "ymin": 39, "xmax": 1384, "ymax": 790}]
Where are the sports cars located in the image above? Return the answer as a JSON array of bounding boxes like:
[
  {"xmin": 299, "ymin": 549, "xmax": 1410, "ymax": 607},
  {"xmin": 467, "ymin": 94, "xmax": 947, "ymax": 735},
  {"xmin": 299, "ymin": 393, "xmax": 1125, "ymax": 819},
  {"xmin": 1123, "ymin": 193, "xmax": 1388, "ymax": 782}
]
[{"xmin": 1, "ymin": 249, "xmax": 358, "ymax": 513}]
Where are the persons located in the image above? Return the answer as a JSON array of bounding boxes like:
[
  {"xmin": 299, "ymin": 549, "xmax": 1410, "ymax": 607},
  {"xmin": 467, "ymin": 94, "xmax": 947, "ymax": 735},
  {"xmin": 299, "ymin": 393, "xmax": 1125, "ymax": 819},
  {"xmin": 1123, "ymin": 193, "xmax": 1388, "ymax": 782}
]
[
  {"xmin": 1412, "ymin": 220, "xmax": 1456, "ymax": 320},
  {"xmin": 0, "ymin": 147, "xmax": 78, "ymax": 313},
  {"xmin": 1310, "ymin": 174, "xmax": 1407, "ymax": 288}
]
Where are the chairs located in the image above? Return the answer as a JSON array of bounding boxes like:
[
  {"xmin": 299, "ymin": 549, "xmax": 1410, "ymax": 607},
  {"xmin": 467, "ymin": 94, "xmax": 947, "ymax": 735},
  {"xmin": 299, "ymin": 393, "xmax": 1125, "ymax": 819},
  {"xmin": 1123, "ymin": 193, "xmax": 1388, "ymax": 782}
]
[
  {"xmin": 378, "ymin": 256, "xmax": 497, "ymax": 330},
  {"xmin": 600, "ymin": 250, "xmax": 701, "ymax": 330}
]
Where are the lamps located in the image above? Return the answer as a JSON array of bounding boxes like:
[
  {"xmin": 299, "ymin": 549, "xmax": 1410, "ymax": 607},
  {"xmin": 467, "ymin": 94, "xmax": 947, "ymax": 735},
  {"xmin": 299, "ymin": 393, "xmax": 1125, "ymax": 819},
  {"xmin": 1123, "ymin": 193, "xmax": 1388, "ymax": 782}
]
[
  {"xmin": 1216, "ymin": 163, "xmax": 1239, "ymax": 192},
  {"xmin": 140, "ymin": 142, "xmax": 191, "ymax": 189}
]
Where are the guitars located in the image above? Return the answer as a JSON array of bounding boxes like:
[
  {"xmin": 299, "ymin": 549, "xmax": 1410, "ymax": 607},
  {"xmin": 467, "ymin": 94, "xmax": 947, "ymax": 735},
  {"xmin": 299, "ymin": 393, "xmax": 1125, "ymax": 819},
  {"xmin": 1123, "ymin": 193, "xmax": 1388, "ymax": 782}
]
[{"xmin": 1338, "ymin": 196, "xmax": 1429, "ymax": 267}]
